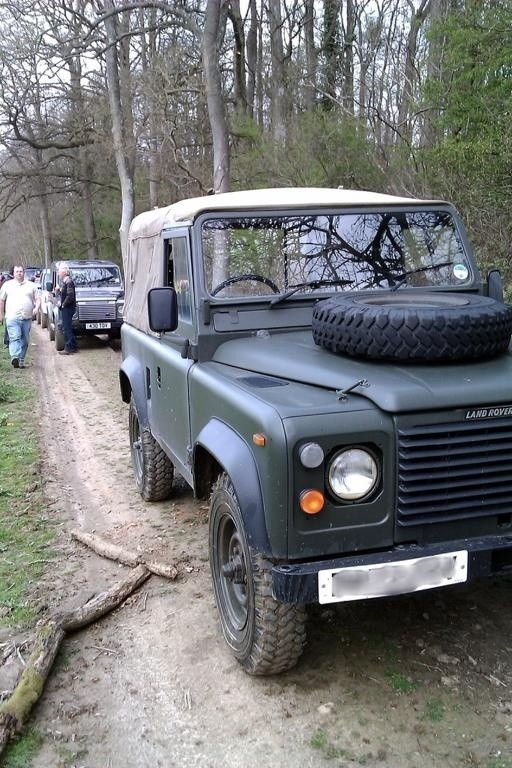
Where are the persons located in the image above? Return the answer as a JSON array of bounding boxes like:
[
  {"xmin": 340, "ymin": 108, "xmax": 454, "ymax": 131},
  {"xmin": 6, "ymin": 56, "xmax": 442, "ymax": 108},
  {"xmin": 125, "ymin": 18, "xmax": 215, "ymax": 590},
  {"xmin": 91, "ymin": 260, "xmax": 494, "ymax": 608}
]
[
  {"xmin": 55, "ymin": 266, "xmax": 79, "ymax": 355},
  {"xmin": 0, "ymin": 273, "xmax": 5, "ymax": 284},
  {"xmin": 0, "ymin": 264, "xmax": 41, "ymax": 368},
  {"xmin": 0, "ymin": 266, "xmax": 14, "ymax": 349}
]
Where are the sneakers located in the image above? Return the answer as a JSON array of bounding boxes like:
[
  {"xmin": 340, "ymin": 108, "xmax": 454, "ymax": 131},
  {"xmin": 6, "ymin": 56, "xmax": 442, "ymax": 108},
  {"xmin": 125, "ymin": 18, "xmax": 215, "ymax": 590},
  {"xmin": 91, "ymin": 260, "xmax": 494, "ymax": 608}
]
[
  {"xmin": 12, "ymin": 359, "xmax": 25, "ymax": 369},
  {"xmin": 58, "ymin": 348, "xmax": 77, "ymax": 355}
]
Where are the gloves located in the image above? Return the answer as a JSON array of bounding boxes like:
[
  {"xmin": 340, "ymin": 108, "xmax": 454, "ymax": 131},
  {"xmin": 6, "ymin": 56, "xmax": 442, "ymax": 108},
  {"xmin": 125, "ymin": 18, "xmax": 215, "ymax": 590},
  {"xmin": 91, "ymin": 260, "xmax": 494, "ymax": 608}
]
[{"xmin": 59, "ymin": 306, "xmax": 64, "ymax": 311}]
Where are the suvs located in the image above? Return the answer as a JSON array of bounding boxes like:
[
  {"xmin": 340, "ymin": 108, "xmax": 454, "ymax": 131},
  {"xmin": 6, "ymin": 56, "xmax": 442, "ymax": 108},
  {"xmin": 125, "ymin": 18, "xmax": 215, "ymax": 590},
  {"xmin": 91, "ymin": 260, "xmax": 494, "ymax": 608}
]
[
  {"xmin": 0, "ymin": 258, "xmax": 127, "ymax": 350},
  {"xmin": 116, "ymin": 188, "xmax": 512, "ymax": 678}
]
